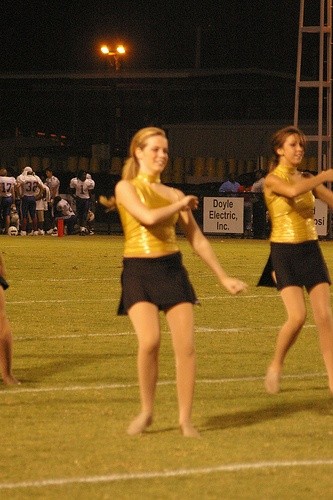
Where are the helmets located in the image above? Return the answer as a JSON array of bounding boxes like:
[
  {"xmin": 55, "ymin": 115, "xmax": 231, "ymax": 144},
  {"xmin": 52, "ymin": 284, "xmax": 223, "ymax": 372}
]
[{"xmin": 7, "ymin": 226, "xmax": 19, "ymax": 236}]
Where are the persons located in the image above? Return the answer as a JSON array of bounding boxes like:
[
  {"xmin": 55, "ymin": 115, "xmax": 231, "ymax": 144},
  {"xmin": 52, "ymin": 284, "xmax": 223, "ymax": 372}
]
[
  {"xmin": 0, "ymin": 256, "xmax": 21, "ymax": 387},
  {"xmin": 114, "ymin": 127, "xmax": 249, "ymax": 437},
  {"xmin": 0, "ymin": 167, "xmax": 267, "ymax": 236},
  {"xmin": 255, "ymin": 128, "xmax": 333, "ymax": 399}
]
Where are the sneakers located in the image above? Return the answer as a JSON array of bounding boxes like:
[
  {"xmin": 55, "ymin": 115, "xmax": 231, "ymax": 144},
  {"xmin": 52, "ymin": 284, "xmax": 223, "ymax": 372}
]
[{"xmin": 20, "ymin": 227, "xmax": 68, "ymax": 236}]
